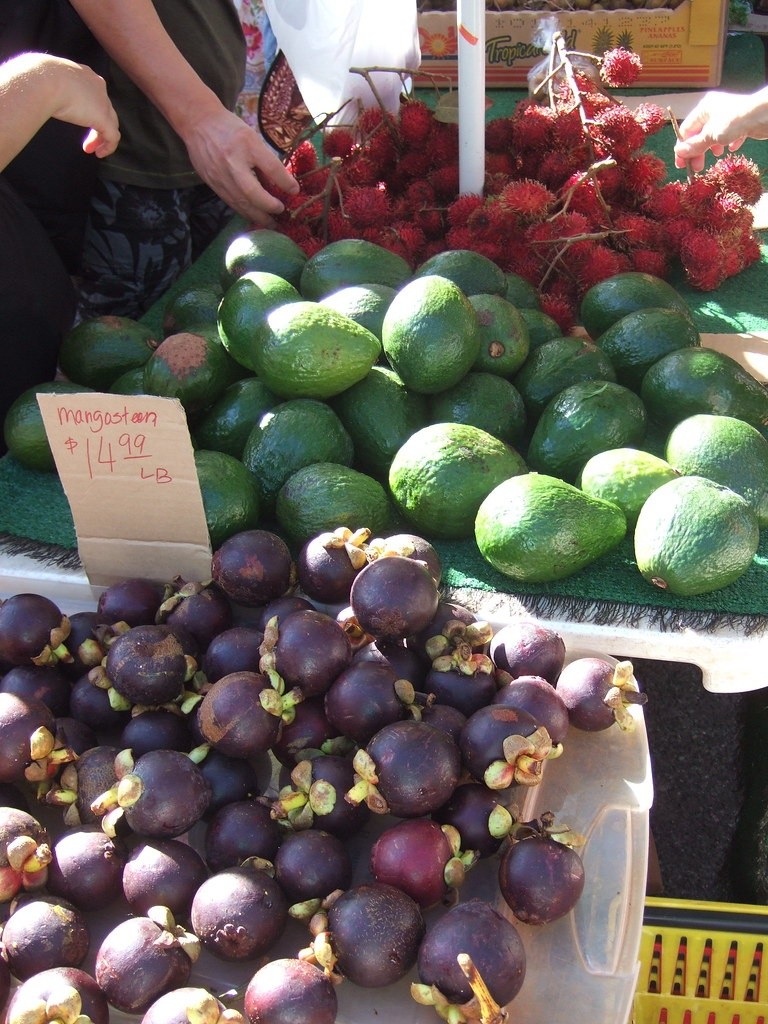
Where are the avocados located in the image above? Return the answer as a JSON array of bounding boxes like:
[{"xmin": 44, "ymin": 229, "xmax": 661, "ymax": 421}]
[{"xmin": 5, "ymin": 229, "xmax": 768, "ymax": 596}]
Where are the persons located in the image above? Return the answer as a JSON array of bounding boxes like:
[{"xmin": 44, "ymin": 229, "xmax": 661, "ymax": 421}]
[
  {"xmin": 45, "ymin": 0, "xmax": 307, "ymax": 333},
  {"xmin": 0, "ymin": 49, "xmax": 123, "ymax": 467},
  {"xmin": 674, "ymin": 87, "xmax": 768, "ymax": 173}
]
